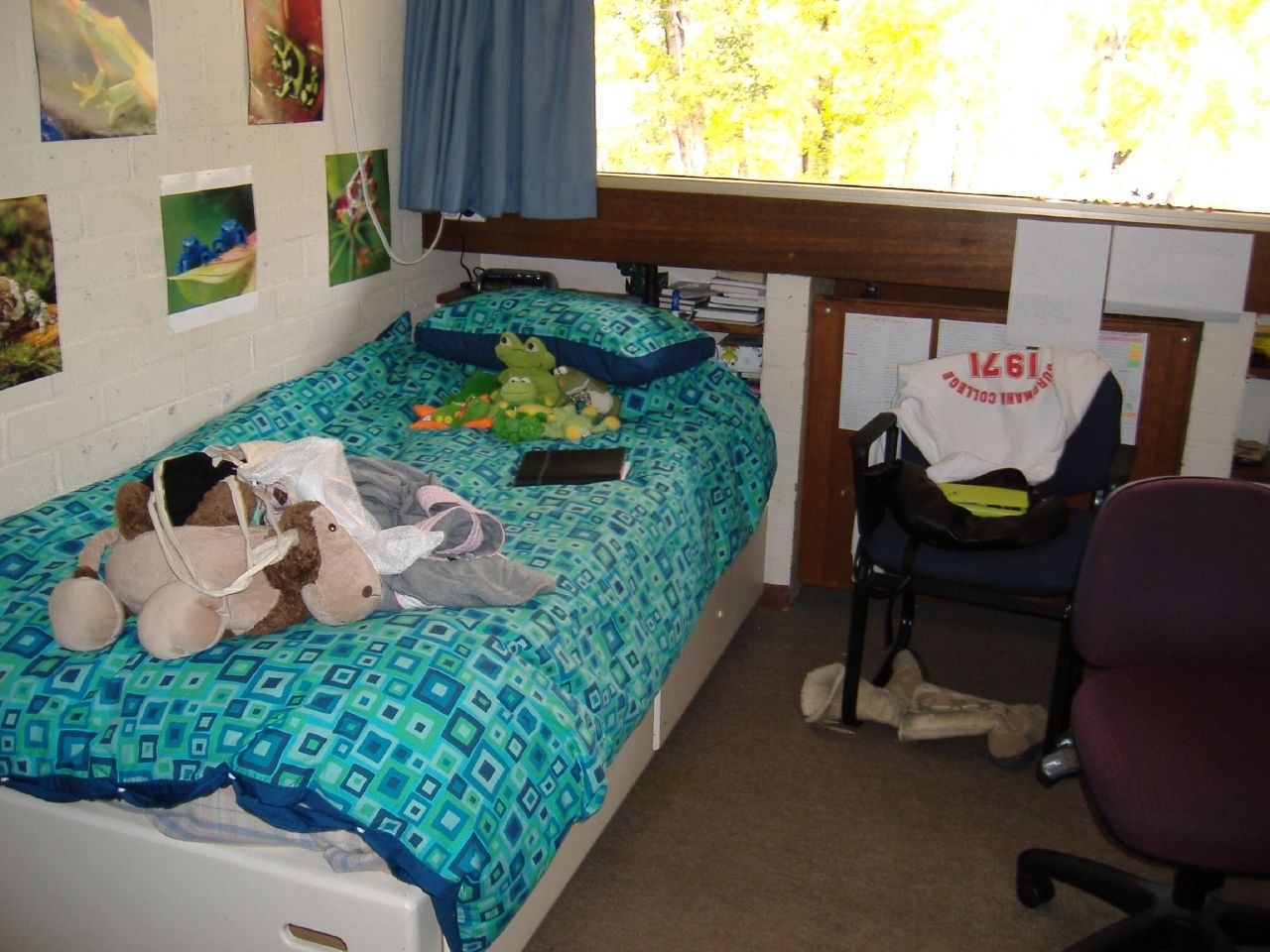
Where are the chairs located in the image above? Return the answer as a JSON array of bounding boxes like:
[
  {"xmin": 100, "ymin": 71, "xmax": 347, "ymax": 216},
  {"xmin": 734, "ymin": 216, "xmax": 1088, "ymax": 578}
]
[
  {"xmin": 838, "ymin": 343, "xmax": 1126, "ymax": 790},
  {"xmin": 1015, "ymin": 474, "xmax": 1270, "ymax": 952}
]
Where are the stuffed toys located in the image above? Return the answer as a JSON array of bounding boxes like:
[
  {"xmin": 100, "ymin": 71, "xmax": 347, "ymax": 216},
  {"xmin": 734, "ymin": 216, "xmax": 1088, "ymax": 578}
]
[
  {"xmin": 45, "ymin": 451, "xmax": 382, "ymax": 660},
  {"xmin": 409, "ymin": 334, "xmax": 620, "ymax": 441}
]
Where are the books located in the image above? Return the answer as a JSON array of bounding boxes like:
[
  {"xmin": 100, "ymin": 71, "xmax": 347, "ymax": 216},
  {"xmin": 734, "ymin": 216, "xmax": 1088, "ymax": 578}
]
[{"xmin": 516, "ymin": 447, "xmax": 630, "ymax": 486}]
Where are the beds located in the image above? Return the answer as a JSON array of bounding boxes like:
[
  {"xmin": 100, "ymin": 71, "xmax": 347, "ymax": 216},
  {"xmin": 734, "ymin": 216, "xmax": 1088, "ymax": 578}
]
[{"xmin": 0, "ymin": 309, "xmax": 777, "ymax": 952}]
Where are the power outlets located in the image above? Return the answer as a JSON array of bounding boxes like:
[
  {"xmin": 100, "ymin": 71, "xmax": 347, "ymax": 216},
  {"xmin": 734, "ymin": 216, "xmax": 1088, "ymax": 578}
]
[{"xmin": 444, "ymin": 213, "xmax": 486, "ymax": 223}]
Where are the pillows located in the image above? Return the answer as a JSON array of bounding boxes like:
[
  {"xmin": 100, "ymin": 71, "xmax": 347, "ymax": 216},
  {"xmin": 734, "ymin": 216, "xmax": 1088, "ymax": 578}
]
[{"xmin": 415, "ymin": 287, "xmax": 716, "ymax": 390}]
